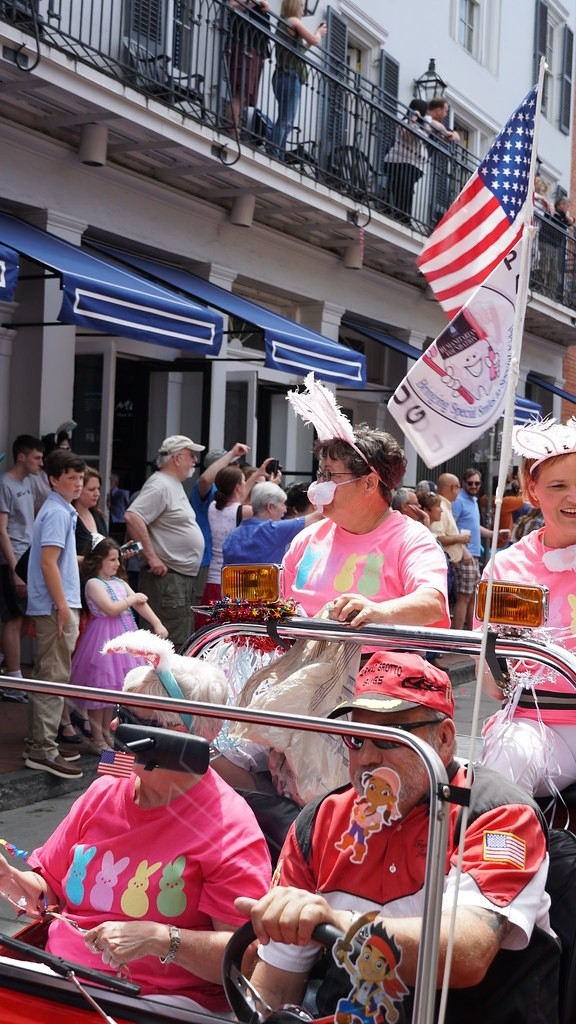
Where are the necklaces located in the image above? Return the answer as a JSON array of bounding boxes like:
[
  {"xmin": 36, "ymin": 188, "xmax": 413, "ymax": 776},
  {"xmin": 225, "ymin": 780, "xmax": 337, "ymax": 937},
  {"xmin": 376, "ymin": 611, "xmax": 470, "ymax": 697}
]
[{"xmin": 97, "ymin": 575, "xmax": 136, "ymax": 631}]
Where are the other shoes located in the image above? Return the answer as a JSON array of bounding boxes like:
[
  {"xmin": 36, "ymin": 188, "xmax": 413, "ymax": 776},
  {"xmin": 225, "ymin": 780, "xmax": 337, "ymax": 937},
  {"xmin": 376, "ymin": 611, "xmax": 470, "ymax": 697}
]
[
  {"xmin": 78, "ymin": 717, "xmax": 94, "ymax": 738},
  {"xmin": 58, "ymin": 724, "xmax": 83, "ymax": 743},
  {"xmin": 90, "ymin": 739, "xmax": 114, "ymax": 757}
]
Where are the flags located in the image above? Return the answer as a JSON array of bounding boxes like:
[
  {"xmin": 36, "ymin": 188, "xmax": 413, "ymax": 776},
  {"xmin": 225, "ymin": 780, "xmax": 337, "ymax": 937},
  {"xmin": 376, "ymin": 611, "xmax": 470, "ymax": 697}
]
[
  {"xmin": 416, "ymin": 84, "xmax": 537, "ymax": 321},
  {"xmin": 97, "ymin": 750, "xmax": 135, "ymax": 778},
  {"xmin": 384, "ymin": 228, "xmax": 530, "ymax": 468}
]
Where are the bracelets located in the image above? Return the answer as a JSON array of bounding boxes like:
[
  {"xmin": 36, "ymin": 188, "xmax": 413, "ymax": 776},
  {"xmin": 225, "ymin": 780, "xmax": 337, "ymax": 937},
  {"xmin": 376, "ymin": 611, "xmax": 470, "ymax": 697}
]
[{"xmin": 159, "ymin": 924, "xmax": 180, "ymax": 964}]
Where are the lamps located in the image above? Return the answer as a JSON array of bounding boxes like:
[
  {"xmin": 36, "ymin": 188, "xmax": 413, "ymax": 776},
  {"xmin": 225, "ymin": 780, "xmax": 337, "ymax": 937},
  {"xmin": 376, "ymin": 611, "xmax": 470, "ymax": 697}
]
[
  {"xmin": 303, "ymin": 0, "xmax": 319, "ymax": 17},
  {"xmin": 231, "ymin": 195, "xmax": 255, "ymax": 227},
  {"xmin": 80, "ymin": 124, "xmax": 107, "ymax": 166},
  {"xmin": 417, "ymin": 58, "xmax": 446, "ymax": 102},
  {"xmin": 344, "ymin": 244, "xmax": 363, "ymax": 269}
]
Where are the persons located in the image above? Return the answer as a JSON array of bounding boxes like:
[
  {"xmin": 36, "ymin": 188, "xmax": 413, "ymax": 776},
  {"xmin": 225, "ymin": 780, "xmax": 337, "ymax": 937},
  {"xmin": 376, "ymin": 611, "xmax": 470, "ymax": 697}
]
[
  {"xmin": 0, "ymin": 425, "xmax": 535, "ymax": 778},
  {"xmin": 268, "ymin": 0, "xmax": 326, "ymax": 159},
  {"xmin": 271, "ymin": 431, "xmax": 450, "ymax": 670},
  {"xmin": 224, "ymin": 0, "xmax": 271, "ymax": 140},
  {"xmin": 0, "ymin": 628, "xmax": 273, "ymax": 1024},
  {"xmin": 471, "ymin": 414, "xmax": 576, "ymax": 800},
  {"xmin": 384, "ymin": 96, "xmax": 460, "ymax": 227},
  {"xmin": 531, "ymin": 176, "xmax": 575, "ymax": 301},
  {"xmin": 232, "ymin": 651, "xmax": 566, "ymax": 1024}
]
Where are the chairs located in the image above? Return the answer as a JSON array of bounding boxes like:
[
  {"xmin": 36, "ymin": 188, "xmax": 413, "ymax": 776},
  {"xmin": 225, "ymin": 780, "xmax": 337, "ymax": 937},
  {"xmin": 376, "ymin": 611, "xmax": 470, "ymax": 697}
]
[
  {"xmin": 255, "ymin": 109, "xmax": 319, "ymax": 179},
  {"xmin": 123, "ymin": 37, "xmax": 207, "ymax": 122},
  {"xmin": 329, "ymin": 146, "xmax": 392, "ymax": 213}
]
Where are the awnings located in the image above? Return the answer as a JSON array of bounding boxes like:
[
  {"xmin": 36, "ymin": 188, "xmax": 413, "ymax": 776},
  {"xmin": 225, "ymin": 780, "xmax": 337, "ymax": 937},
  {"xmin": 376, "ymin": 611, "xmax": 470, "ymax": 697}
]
[
  {"xmin": 88, "ymin": 241, "xmax": 367, "ymax": 389},
  {"xmin": 0, "ymin": 213, "xmax": 221, "ymax": 357},
  {"xmin": 341, "ymin": 322, "xmax": 576, "ymax": 427}
]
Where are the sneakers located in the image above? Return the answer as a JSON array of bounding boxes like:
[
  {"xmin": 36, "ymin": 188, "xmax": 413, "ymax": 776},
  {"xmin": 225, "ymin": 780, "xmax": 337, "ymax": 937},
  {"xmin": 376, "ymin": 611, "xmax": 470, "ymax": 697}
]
[
  {"xmin": 26, "ymin": 753, "xmax": 84, "ymax": 779},
  {"xmin": 23, "ymin": 740, "xmax": 80, "ymax": 761}
]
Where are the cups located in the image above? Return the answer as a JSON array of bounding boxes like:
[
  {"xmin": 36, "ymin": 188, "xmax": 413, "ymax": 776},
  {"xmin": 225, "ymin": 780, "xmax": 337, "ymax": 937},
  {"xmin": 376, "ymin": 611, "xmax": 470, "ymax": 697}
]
[{"xmin": 461, "ymin": 529, "xmax": 471, "ymax": 535}]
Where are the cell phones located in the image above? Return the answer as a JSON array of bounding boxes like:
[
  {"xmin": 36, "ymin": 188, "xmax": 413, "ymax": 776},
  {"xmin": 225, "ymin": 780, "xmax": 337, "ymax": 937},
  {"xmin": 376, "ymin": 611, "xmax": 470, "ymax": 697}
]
[
  {"xmin": 120, "ymin": 541, "xmax": 143, "ymax": 556},
  {"xmin": 270, "ymin": 460, "xmax": 279, "ymax": 475}
]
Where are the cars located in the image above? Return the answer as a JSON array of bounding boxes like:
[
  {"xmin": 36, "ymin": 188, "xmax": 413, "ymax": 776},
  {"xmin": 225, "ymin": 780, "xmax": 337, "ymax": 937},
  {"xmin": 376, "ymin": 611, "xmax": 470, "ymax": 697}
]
[{"xmin": 1, "ymin": 560, "xmax": 575, "ymax": 1024}]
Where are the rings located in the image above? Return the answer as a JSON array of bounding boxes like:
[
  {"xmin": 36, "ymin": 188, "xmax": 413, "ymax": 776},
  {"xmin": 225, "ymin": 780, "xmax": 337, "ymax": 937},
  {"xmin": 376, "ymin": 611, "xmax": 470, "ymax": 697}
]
[{"xmin": 92, "ymin": 943, "xmax": 102, "ymax": 953}]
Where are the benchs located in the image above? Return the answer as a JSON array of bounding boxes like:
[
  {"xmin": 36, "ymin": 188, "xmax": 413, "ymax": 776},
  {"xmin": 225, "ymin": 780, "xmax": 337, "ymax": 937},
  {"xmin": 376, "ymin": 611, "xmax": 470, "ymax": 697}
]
[{"xmin": 227, "ymin": 786, "xmax": 576, "ymax": 1024}]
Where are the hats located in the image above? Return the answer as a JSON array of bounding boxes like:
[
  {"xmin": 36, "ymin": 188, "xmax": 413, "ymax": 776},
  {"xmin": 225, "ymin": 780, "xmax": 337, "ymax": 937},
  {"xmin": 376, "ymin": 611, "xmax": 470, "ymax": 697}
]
[
  {"xmin": 326, "ymin": 649, "xmax": 453, "ymax": 729},
  {"xmin": 158, "ymin": 434, "xmax": 206, "ymax": 459}
]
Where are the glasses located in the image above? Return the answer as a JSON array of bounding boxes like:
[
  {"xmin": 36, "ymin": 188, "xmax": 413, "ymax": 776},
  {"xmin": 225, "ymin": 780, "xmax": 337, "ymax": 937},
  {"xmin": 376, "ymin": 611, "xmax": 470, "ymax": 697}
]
[
  {"xmin": 341, "ymin": 717, "xmax": 444, "ymax": 750},
  {"xmin": 316, "ymin": 467, "xmax": 360, "ymax": 482},
  {"xmin": 467, "ymin": 481, "xmax": 482, "ymax": 487},
  {"xmin": 115, "ymin": 703, "xmax": 182, "ymax": 728}
]
